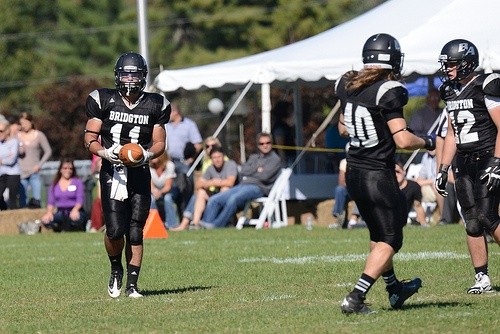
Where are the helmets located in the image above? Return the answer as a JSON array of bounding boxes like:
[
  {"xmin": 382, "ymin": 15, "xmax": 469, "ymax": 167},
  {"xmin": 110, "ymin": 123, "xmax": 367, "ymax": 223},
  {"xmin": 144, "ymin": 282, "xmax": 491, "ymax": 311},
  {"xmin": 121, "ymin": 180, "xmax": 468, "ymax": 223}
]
[
  {"xmin": 439, "ymin": 39, "xmax": 479, "ymax": 81},
  {"xmin": 113, "ymin": 53, "xmax": 147, "ymax": 95},
  {"xmin": 362, "ymin": 33, "xmax": 401, "ymax": 73}
]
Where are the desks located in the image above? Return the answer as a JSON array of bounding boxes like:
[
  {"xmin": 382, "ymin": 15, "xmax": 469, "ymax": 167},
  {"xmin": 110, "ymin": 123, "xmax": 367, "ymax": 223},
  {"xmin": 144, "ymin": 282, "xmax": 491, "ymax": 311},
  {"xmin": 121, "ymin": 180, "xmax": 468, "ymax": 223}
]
[{"xmin": 285, "ymin": 174, "xmax": 339, "ymax": 201}]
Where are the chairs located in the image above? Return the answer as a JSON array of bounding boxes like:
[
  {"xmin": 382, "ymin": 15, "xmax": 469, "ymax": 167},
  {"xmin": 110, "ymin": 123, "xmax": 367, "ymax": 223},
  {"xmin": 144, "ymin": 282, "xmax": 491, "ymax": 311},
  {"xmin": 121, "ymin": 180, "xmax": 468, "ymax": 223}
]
[
  {"xmin": 405, "ymin": 163, "xmax": 422, "ymax": 224},
  {"xmin": 236, "ymin": 167, "xmax": 293, "ymax": 232}
]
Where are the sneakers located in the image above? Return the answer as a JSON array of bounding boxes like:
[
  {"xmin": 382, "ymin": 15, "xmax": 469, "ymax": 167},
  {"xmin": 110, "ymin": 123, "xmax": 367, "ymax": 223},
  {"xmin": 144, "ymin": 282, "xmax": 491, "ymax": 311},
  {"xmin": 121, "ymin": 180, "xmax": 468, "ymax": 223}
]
[
  {"xmin": 467, "ymin": 271, "xmax": 494, "ymax": 293},
  {"xmin": 108, "ymin": 269, "xmax": 123, "ymax": 298},
  {"xmin": 388, "ymin": 278, "xmax": 422, "ymax": 309},
  {"xmin": 341, "ymin": 293, "xmax": 371, "ymax": 315},
  {"xmin": 125, "ymin": 286, "xmax": 143, "ymax": 297}
]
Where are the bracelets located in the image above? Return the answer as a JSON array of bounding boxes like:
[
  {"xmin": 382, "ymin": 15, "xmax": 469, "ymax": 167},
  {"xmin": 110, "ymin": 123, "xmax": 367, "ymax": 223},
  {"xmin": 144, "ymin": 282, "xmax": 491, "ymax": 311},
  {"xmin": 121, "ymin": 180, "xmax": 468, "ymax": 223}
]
[{"xmin": 87, "ymin": 140, "xmax": 99, "ymax": 150}]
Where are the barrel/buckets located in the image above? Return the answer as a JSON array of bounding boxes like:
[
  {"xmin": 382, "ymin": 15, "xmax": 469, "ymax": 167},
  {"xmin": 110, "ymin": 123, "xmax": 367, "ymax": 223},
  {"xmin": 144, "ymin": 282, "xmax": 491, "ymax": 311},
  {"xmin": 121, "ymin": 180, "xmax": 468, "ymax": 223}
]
[{"xmin": 16, "ymin": 221, "xmax": 42, "ymax": 235}]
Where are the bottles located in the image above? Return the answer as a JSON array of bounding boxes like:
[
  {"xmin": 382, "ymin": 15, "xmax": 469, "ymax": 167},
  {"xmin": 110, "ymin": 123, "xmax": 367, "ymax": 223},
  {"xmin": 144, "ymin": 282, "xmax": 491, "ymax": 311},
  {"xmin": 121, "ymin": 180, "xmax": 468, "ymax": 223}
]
[
  {"xmin": 263, "ymin": 217, "xmax": 273, "ymax": 228},
  {"xmin": 306, "ymin": 217, "xmax": 313, "ymax": 231}
]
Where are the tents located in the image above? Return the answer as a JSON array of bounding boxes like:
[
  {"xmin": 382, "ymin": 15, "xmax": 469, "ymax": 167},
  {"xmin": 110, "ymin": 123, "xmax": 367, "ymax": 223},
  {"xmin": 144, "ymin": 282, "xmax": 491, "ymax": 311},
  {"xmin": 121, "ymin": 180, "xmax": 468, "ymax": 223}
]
[{"xmin": 154, "ymin": 0, "xmax": 500, "ymax": 92}]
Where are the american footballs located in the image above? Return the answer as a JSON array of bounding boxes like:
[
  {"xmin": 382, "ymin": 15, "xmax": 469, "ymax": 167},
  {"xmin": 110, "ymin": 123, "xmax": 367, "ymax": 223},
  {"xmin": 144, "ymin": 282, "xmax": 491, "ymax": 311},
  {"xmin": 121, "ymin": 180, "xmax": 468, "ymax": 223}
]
[{"xmin": 118, "ymin": 143, "xmax": 144, "ymax": 166}]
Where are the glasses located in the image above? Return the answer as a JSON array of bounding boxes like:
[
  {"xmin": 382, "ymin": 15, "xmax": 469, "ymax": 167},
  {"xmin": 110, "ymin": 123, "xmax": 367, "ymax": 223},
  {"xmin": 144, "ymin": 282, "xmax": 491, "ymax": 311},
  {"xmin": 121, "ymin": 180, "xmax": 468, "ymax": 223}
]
[
  {"xmin": 0, "ymin": 130, "xmax": 4, "ymax": 133},
  {"xmin": 259, "ymin": 142, "xmax": 271, "ymax": 145}
]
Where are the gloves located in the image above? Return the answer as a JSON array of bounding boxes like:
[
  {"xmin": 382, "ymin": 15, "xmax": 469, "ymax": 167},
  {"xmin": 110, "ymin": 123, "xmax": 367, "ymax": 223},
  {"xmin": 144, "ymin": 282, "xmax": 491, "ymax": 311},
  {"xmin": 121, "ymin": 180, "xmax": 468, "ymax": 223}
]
[
  {"xmin": 97, "ymin": 144, "xmax": 121, "ymax": 163},
  {"xmin": 132, "ymin": 144, "xmax": 154, "ymax": 166},
  {"xmin": 435, "ymin": 164, "xmax": 450, "ymax": 197},
  {"xmin": 479, "ymin": 157, "xmax": 500, "ymax": 191}
]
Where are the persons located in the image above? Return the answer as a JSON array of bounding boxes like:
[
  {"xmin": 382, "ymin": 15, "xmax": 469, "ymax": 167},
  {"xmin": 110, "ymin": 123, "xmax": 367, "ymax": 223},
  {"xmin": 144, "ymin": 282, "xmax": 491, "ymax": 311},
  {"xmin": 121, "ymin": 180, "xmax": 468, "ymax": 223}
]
[
  {"xmin": 335, "ymin": 33, "xmax": 436, "ymax": 312},
  {"xmin": 149, "ymin": 103, "xmax": 239, "ymax": 231},
  {"xmin": 42, "ymin": 158, "xmax": 87, "ymax": 231},
  {"xmin": 85, "ymin": 53, "xmax": 171, "ymax": 299},
  {"xmin": 394, "ymin": 83, "xmax": 461, "ymax": 227},
  {"xmin": 0, "ymin": 112, "xmax": 51, "ymax": 210},
  {"xmin": 189, "ymin": 132, "xmax": 282, "ymax": 231},
  {"xmin": 328, "ymin": 142, "xmax": 360, "ymax": 228},
  {"xmin": 436, "ymin": 39, "xmax": 500, "ymax": 294}
]
